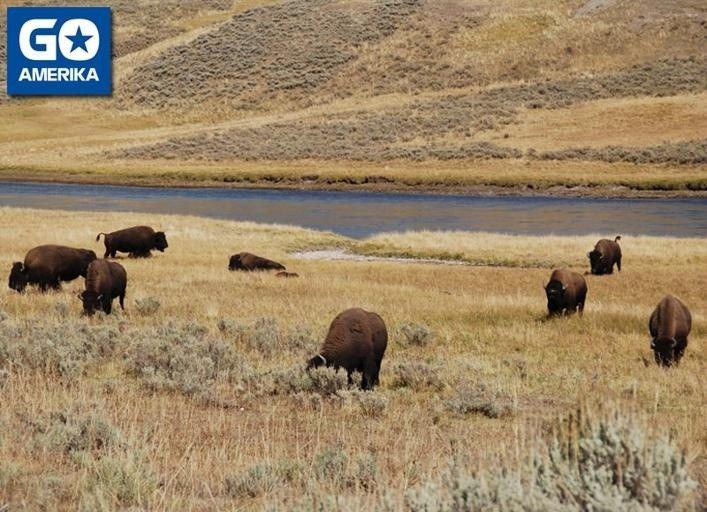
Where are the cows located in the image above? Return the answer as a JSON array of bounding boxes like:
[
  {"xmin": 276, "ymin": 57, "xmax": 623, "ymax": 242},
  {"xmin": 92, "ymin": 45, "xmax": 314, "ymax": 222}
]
[
  {"xmin": 95, "ymin": 225, "xmax": 168, "ymax": 259},
  {"xmin": 585, "ymin": 235, "xmax": 622, "ymax": 275},
  {"xmin": 304, "ymin": 307, "xmax": 388, "ymax": 391},
  {"xmin": 227, "ymin": 252, "xmax": 286, "ymax": 271},
  {"xmin": 542, "ymin": 269, "xmax": 588, "ymax": 320},
  {"xmin": 77, "ymin": 259, "xmax": 127, "ymax": 317},
  {"xmin": 8, "ymin": 245, "xmax": 97, "ymax": 294},
  {"xmin": 275, "ymin": 271, "xmax": 299, "ymax": 278},
  {"xmin": 648, "ymin": 294, "xmax": 692, "ymax": 368}
]
